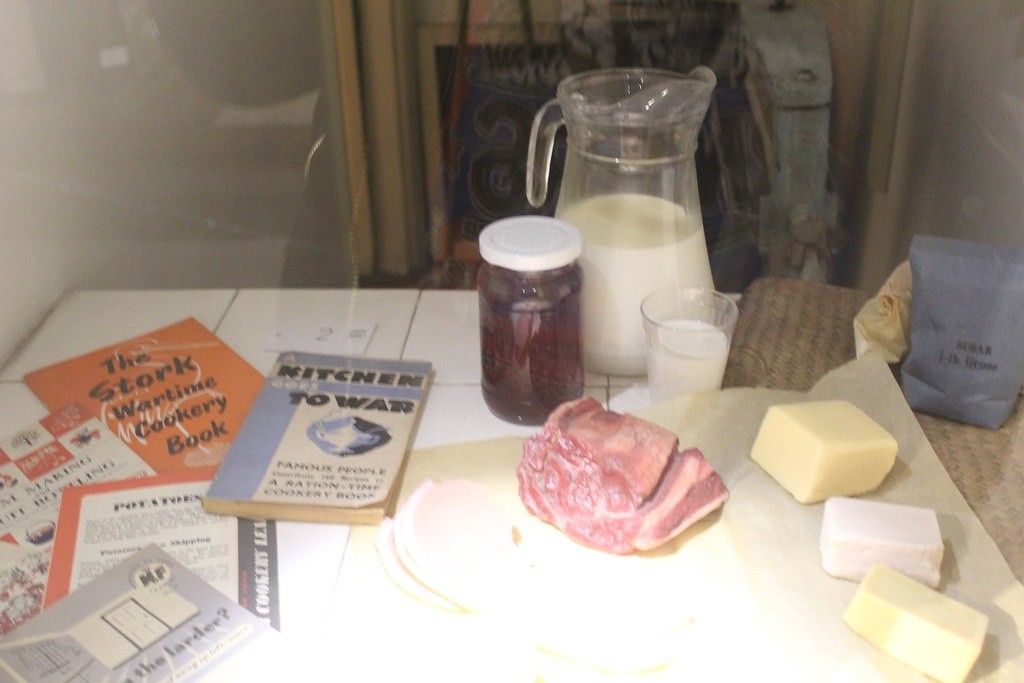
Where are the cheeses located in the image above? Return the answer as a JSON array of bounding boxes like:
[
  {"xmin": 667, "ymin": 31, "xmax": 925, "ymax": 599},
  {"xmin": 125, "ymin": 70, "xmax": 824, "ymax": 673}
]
[
  {"xmin": 844, "ymin": 563, "xmax": 989, "ymax": 683},
  {"xmin": 751, "ymin": 400, "xmax": 898, "ymax": 504},
  {"xmin": 818, "ymin": 496, "xmax": 945, "ymax": 589}
]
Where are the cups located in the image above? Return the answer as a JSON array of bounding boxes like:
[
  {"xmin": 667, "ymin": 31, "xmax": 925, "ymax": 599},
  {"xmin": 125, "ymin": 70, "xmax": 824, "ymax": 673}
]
[{"xmin": 639, "ymin": 287, "xmax": 738, "ymax": 406}]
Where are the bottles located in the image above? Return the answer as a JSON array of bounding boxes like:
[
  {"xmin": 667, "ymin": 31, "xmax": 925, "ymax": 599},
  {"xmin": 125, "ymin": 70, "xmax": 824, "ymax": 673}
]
[{"xmin": 476, "ymin": 215, "xmax": 585, "ymax": 427}]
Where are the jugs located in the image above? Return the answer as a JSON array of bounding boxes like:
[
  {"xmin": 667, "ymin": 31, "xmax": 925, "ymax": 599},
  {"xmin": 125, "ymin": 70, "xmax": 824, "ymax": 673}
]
[{"xmin": 526, "ymin": 66, "xmax": 716, "ymax": 376}]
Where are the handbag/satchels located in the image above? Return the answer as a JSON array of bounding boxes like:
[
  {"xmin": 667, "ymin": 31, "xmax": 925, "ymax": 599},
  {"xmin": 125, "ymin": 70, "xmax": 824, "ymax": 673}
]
[{"xmin": 897, "ymin": 236, "xmax": 1024, "ymax": 433}]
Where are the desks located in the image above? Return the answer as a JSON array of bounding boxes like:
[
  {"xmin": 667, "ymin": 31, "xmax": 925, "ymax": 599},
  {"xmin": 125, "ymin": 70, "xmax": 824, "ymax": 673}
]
[{"xmin": 0, "ymin": 289, "xmax": 1024, "ymax": 683}]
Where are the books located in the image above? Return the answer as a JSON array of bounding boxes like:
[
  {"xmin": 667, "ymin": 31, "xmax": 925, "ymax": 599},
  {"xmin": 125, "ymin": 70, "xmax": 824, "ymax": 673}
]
[
  {"xmin": 1, "ymin": 541, "xmax": 266, "ymax": 683},
  {"xmin": 201, "ymin": 349, "xmax": 433, "ymax": 526}
]
